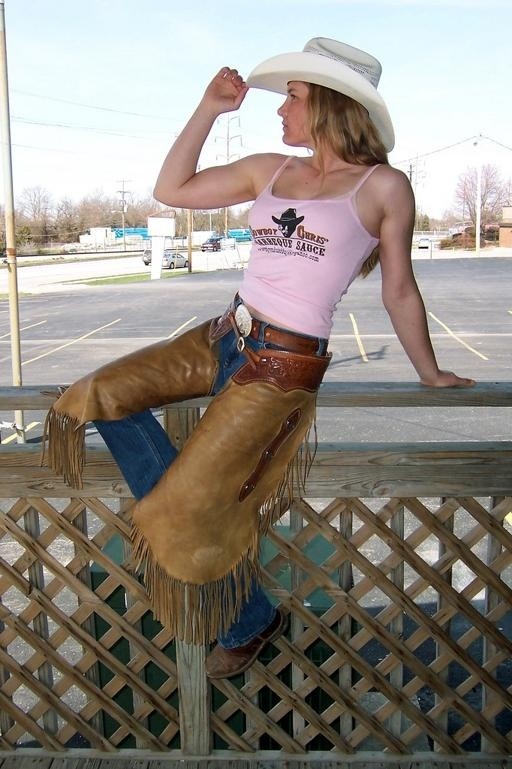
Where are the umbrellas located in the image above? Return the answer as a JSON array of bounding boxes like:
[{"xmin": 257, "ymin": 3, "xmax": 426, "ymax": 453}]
[{"xmin": 204, "ymin": 607, "xmax": 288, "ymax": 679}]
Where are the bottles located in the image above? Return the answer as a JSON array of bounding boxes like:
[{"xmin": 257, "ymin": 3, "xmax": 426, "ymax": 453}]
[{"xmin": 245, "ymin": 37, "xmax": 394, "ymax": 153}]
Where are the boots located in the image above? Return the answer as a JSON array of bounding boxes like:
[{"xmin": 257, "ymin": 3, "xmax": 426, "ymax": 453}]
[{"xmin": 200, "ymin": 237, "xmax": 226, "ymax": 252}]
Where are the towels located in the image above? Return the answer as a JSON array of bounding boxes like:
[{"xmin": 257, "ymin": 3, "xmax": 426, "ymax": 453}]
[{"xmin": 76, "ymin": 525, "xmax": 357, "ymax": 751}]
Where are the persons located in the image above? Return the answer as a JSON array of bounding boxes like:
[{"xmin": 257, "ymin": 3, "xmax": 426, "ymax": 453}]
[{"xmin": 40, "ymin": 36, "xmax": 476, "ymax": 680}]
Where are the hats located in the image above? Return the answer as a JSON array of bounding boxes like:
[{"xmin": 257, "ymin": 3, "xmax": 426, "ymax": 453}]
[
  {"xmin": 161, "ymin": 253, "xmax": 188, "ymax": 268},
  {"xmin": 416, "ymin": 239, "xmax": 432, "ymax": 249}
]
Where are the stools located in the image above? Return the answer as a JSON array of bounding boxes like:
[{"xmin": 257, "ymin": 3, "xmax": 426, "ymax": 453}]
[
  {"xmin": 235, "ymin": 304, "xmax": 318, "ymax": 356},
  {"xmin": 228, "ymin": 314, "xmax": 260, "ymax": 372}
]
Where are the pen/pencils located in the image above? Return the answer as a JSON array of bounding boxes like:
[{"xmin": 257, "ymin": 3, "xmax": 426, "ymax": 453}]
[{"xmin": 142, "ymin": 249, "xmax": 167, "ymax": 265}]
